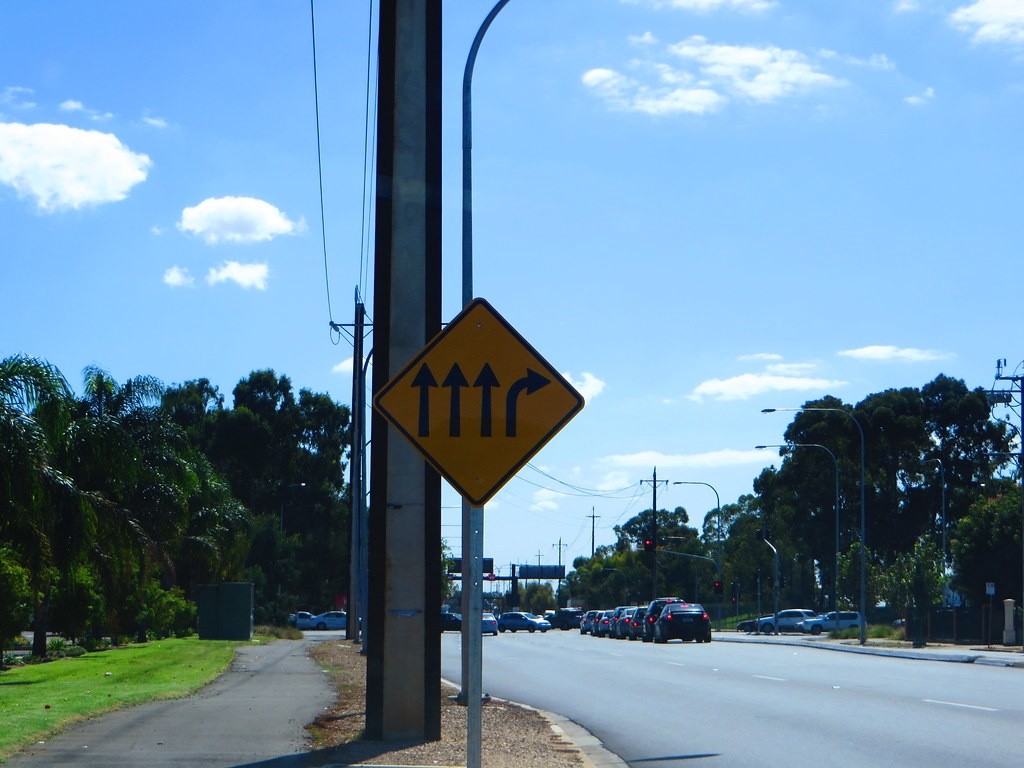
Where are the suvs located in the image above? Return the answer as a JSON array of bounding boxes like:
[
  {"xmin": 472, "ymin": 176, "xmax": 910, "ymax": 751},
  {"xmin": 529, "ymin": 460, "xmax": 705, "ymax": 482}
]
[{"xmin": 639, "ymin": 598, "xmax": 687, "ymax": 643}]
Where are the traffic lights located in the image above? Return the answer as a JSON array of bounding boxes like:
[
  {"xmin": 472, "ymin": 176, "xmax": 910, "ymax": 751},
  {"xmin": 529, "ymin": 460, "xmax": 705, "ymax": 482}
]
[
  {"xmin": 714, "ymin": 581, "xmax": 720, "ymax": 593},
  {"xmin": 768, "ymin": 529, "xmax": 772, "ymax": 540},
  {"xmin": 644, "ymin": 539, "xmax": 654, "ymax": 551}
]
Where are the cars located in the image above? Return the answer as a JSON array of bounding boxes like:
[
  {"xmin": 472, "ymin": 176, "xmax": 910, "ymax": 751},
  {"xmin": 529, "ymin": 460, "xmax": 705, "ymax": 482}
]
[
  {"xmin": 482, "ymin": 613, "xmax": 498, "ymax": 636},
  {"xmin": 607, "ymin": 607, "xmax": 628, "ymax": 638},
  {"xmin": 615, "ymin": 609, "xmax": 636, "ymax": 640},
  {"xmin": 310, "ymin": 611, "xmax": 362, "ymax": 631},
  {"xmin": 577, "ymin": 610, "xmax": 599, "ymax": 636},
  {"xmin": 653, "ymin": 604, "xmax": 712, "ymax": 644},
  {"xmin": 545, "ymin": 608, "xmax": 586, "ymax": 631},
  {"xmin": 589, "ymin": 611, "xmax": 606, "ymax": 637},
  {"xmin": 626, "ymin": 606, "xmax": 649, "ymax": 642},
  {"xmin": 597, "ymin": 610, "xmax": 614, "ymax": 639},
  {"xmin": 499, "ymin": 612, "xmax": 551, "ymax": 634},
  {"xmin": 737, "ymin": 609, "xmax": 861, "ymax": 637},
  {"xmin": 440, "ymin": 613, "xmax": 463, "ymax": 632}
]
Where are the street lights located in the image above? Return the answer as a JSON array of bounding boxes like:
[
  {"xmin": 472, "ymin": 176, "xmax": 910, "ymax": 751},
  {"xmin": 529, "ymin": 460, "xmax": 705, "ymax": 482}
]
[
  {"xmin": 755, "ymin": 407, "xmax": 866, "ymax": 646},
  {"xmin": 675, "ymin": 482, "xmax": 722, "ymax": 633}
]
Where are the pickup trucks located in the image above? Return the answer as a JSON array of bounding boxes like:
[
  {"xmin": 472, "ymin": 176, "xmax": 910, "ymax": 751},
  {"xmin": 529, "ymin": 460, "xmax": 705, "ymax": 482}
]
[{"xmin": 288, "ymin": 611, "xmax": 316, "ymax": 628}]
